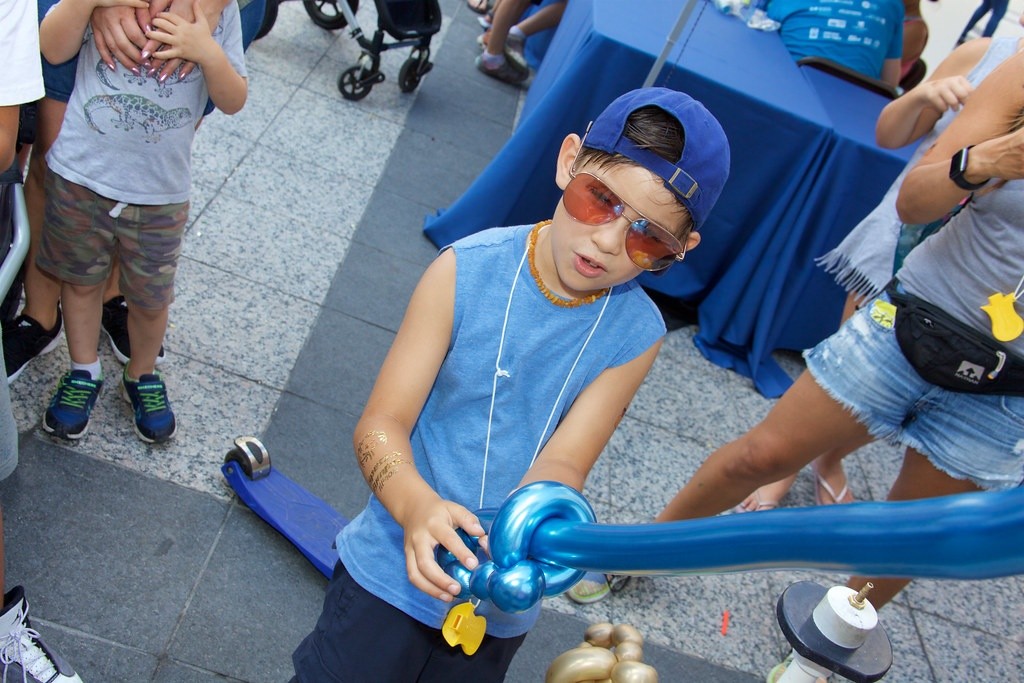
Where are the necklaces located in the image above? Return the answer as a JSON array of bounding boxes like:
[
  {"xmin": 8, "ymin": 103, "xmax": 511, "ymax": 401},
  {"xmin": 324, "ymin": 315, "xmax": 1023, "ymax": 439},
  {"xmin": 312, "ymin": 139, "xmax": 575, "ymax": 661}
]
[
  {"xmin": 440, "ymin": 217, "xmax": 615, "ymax": 657},
  {"xmin": 980, "ymin": 276, "xmax": 1024, "ymax": 343}
]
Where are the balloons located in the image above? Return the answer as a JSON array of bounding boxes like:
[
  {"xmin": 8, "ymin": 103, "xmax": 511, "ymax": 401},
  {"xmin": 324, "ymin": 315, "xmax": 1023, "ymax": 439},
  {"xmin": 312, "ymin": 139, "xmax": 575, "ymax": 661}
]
[{"xmin": 439, "ymin": 480, "xmax": 1023, "ymax": 615}]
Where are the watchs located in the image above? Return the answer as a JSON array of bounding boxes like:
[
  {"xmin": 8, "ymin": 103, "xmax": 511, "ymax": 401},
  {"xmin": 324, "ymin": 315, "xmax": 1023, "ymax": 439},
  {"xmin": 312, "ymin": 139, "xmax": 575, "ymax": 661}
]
[{"xmin": 949, "ymin": 145, "xmax": 990, "ymax": 191}]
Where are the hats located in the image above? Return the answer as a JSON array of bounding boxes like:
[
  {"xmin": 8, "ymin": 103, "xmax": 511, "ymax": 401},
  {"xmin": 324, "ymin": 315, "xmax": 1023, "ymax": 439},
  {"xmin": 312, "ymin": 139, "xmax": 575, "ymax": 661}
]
[{"xmin": 583, "ymin": 86, "xmax": 732, "ymax": 233}]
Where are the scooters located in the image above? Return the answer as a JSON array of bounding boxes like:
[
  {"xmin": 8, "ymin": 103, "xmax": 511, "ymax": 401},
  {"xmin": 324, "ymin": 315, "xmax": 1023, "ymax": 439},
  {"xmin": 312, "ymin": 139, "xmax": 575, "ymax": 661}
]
[{"xmin": 221, "ymin": 435, "xmax": 352, "ymax": 581}]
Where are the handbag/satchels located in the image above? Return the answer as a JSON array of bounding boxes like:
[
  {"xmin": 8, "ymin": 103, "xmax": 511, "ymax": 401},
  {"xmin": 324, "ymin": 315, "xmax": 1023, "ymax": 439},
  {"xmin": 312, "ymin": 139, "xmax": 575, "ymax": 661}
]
[{"xmin": 886, "ymin": 278, "xmax": 1024, "ymax": 395}]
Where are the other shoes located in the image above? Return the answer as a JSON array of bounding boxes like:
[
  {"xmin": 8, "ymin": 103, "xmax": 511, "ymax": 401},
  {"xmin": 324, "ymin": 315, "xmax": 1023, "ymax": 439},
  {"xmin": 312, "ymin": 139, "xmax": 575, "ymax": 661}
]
[{"xmin": 468, "ymin": 0, "xmax": 530, "ymax": 83}]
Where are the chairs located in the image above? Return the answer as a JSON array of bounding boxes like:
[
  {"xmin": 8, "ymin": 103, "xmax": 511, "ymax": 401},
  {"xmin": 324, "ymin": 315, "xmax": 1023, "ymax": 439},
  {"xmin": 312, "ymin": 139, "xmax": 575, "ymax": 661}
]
[
  {"xmin": 795, "ymin": 56, "xmax": 900, "ymax": 101},
  {"xmin": 899, "ymin": 59, "xmax": 928, "ymax": 98}
]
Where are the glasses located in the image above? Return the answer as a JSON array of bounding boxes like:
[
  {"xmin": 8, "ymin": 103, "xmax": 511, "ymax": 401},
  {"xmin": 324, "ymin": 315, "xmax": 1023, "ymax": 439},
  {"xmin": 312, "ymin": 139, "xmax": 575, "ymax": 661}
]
[{"xmin": 561, "ymin": 121, "xmax": 688, "ymax": 273}]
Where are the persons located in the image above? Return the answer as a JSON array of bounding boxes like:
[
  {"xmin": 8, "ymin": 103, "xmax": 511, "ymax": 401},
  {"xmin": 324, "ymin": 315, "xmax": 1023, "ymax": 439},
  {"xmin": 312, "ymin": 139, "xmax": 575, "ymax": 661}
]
[
  {"xmin": 564, "ymin": 18, "xmax": 1023, "ymax": 683},
  {"xmin": 2, "ymin": 0, "xmax": 247, "ymax": 444},
  {"xmin": 0, "ymin": 0, "xmax": 80, "ymax": 683},
  {"xmin": 284, "ymin": 87, "xmax": 734, "ymax": 683},
  {"xmin": 719, "ymin": 0, "xmax": 929, "ymax": 85},
  {"xmin": 468, "ymin": 0, "xmax": 569, "ymax": 87}
]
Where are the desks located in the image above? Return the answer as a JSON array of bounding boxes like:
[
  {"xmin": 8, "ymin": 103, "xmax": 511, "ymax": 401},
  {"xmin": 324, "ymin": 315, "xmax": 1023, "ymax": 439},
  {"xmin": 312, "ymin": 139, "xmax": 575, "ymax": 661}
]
[{"xmin": 423, "ymin": 1, "xmax": 931, "ymax": 401}]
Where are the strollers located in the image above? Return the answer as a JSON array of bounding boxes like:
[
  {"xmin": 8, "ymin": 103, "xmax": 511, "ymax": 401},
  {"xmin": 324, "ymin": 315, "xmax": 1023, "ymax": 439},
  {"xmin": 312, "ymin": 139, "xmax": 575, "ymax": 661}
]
[{"xmin": 252, "ymin": 1, "xmax": 442, "ymax": 101}]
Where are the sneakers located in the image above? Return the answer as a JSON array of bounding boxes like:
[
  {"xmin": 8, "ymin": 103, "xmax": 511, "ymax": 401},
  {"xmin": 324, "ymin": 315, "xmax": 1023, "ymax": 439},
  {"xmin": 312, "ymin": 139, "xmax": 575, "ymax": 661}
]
[
  {"xmin": 3, "ymin": 300, "xmax": 63, "ymax": 386},
  {"xmin": 102, "ymin": 295, "xmax": 166, "ymax": 364},
  {"xmin": 0, "ymin": 584, "xmax": 84, "ymax": 683},
  {"xmin": 43, "ymin": 362, "xmax": 103, "ymax": 440},
  {"xmin": 565, "ymin": 570, "xmax": 629, "ymax": 604},
  {"xmin": 122, "ymin": 360, "xmax": 177, "ymax": 443}
]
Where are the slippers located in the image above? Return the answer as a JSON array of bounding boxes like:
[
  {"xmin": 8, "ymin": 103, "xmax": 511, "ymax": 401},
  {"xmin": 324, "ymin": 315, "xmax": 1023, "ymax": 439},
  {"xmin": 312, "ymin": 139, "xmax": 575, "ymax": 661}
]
[
  {"xmin": 814, "ymin": 461, "xmax": 854, "ymax": 505},
  {"xmin": 735, "ymin": 475, "xmax": 797, "ymax": 513}
]
